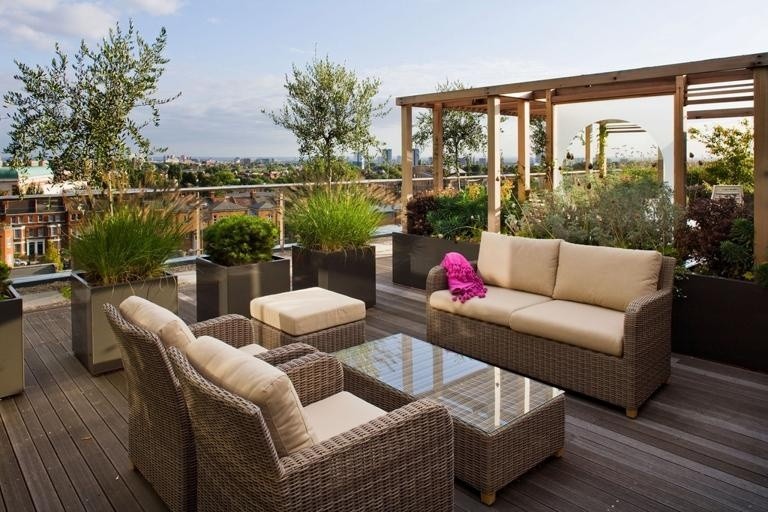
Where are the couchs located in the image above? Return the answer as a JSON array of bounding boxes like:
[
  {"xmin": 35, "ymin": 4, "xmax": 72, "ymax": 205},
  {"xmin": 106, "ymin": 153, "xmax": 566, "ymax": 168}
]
[{"xmin": 425, "ymin": 230, "xmax": 678, "ymax": 418}]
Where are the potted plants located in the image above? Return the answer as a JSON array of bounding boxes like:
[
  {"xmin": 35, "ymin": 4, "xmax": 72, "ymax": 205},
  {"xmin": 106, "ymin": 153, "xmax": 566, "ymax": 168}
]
[
  {"xmin": 0, "ymin": 262, "xmax": 25, "ymax": 400},
  {"xmin": 392, "ymin": 180, "xmax": 767, "ymax": 374}
]
